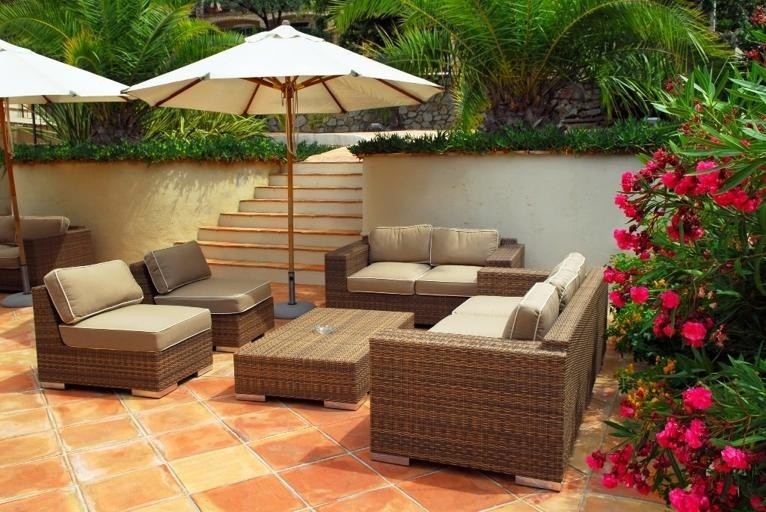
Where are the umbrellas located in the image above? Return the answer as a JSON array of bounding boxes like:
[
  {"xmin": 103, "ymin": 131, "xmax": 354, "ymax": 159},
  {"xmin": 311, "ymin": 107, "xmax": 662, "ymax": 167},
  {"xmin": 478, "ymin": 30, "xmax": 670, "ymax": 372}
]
[
  {"xmin": 120, "ymin": 21, "xmax": 446, "ymax": 303},
  {"xmin": 0, "ymin": 39, "xmax": 140, "ymax": 295}
]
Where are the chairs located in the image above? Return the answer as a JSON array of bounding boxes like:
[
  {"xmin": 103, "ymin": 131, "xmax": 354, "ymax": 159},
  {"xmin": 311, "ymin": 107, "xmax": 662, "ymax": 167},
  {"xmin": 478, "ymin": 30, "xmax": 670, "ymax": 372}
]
[
  {"xmin": 2, "ymin": 212, "xmax": 91, "ymax": 295},
  {"xmin": 28, "ymin": 242, "xmax": 277, "ymax": 401}
]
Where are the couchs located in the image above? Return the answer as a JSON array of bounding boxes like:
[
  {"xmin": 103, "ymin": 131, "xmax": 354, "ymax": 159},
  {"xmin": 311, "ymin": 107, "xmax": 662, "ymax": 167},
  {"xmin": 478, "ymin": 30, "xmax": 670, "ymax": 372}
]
[
  {"xmin": 366, "ymin": 252, "xmax": 613, "ymax": 492},
  {"xmin": 325, "ymin": 223, "xmax": 525, "ymax": 325}
]
